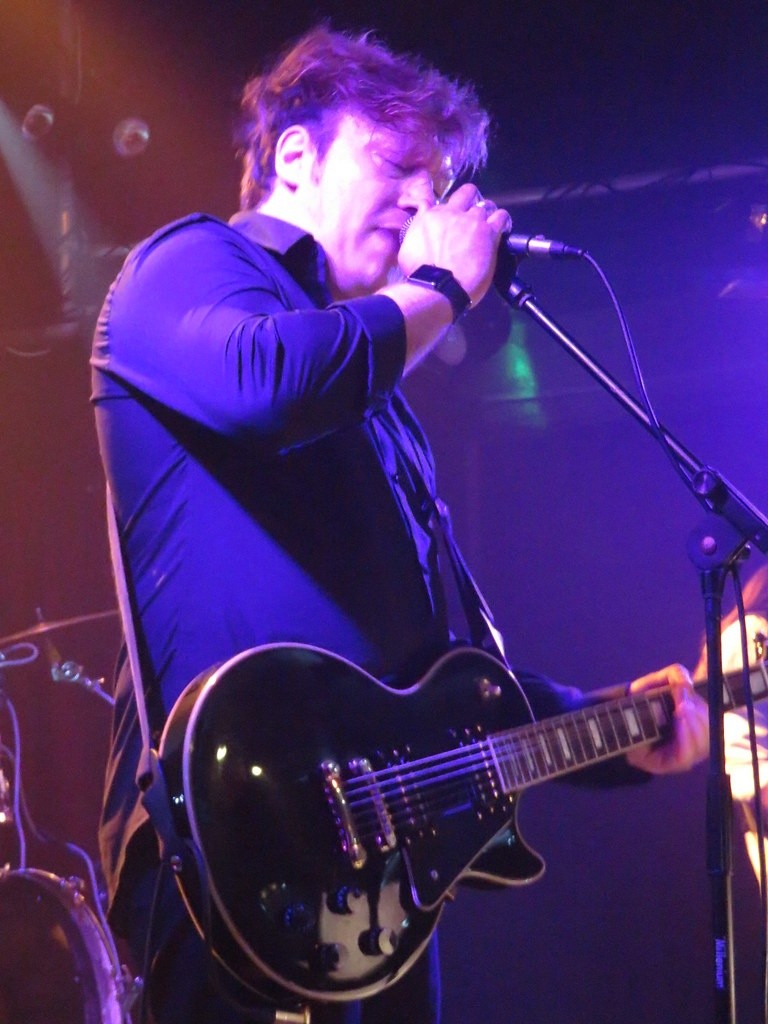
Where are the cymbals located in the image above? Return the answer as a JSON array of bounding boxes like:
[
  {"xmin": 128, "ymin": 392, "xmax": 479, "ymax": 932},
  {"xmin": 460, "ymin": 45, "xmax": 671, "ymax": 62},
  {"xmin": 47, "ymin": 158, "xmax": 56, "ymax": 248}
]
[{"xmin": 0, "ymin": 610, "xmax": 122, "ymax": 642}]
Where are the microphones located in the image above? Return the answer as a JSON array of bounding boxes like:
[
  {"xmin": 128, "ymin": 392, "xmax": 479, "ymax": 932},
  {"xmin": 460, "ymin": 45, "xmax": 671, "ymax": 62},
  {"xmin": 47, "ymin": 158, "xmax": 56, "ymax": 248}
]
[{"xmin": 398, "ymin": 213, "xmax": 587, "ymax": 268}]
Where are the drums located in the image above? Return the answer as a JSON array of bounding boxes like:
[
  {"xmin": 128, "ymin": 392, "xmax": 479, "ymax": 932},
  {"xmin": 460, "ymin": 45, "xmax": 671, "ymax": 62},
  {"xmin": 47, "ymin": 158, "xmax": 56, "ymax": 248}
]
[{"xmin": 1, "ymin": 863, "xmax": 129, "ymax": 1024}]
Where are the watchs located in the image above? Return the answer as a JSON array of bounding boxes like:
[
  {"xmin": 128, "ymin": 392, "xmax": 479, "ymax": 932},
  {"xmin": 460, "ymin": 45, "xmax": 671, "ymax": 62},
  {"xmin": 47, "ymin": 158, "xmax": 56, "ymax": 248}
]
[{"xmin": 403, "ymin": 264, "xmax": 472, "ymax": 324}]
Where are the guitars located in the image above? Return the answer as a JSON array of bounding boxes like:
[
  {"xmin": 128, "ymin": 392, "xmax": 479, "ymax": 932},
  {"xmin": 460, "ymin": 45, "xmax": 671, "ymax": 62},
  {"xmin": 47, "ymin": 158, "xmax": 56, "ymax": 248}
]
[{"xmin": 152, "ymin": 638, "xmax": 767, "ymax": 1010}]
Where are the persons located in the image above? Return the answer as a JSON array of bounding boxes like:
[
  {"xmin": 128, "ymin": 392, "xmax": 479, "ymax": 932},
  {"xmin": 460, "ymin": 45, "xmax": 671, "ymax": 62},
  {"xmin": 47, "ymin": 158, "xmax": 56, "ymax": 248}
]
[{"xmin": 86, "ymin": 25, "xmax": 709, "ymax": 1024}]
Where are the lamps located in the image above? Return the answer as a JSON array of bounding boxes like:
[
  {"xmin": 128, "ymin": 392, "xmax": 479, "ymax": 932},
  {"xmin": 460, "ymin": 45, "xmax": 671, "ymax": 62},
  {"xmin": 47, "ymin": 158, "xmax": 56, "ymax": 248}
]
[
  {"xmin": 23, "ymin": 104, "xmax": 55, "ymax": 141},
  {"xmin": 113, "ymin": 118, "xmax": 151, "ymax": 154}
]
